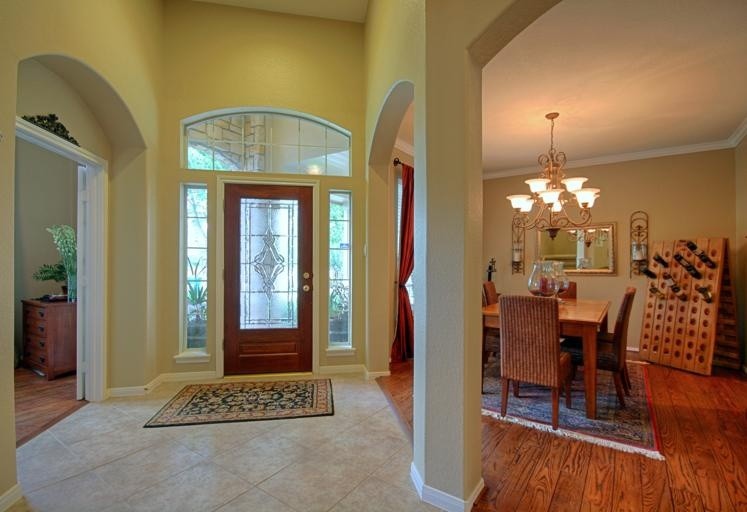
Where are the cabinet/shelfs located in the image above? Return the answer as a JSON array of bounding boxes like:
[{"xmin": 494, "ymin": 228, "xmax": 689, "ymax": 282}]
[{"xmin": 20, "ymin": 299, "xmax": 76, "ymax": 381}]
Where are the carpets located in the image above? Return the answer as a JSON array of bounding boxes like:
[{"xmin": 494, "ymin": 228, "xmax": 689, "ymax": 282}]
[
  {"xmin": 480, "ymin": 359, "xmax": 667, "ymax": 462},
  {"xmin": 142, "ymin": 377, "xmax": 335, "ymax": 430}
]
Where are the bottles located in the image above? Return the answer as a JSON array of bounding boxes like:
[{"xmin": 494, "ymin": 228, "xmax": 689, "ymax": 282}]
[{"xmin": 638, "ymin": 241, "xmax": 716, "ymax": 304}]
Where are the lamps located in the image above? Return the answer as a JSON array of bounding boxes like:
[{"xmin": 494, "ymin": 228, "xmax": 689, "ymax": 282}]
[{"xmin": 506, "ymin": 112, "xmax": 601, "ymax": 241}]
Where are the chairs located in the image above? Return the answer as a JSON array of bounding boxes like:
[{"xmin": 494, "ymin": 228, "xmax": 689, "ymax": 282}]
[{"xmin": 483, "ymin": 279, "xmax": 637, "ymax": 432}]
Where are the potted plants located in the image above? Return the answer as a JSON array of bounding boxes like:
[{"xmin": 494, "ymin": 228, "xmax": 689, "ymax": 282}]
[{"xmin": 31, "ymin": 224, "xmax": 77, "ymax": 305}]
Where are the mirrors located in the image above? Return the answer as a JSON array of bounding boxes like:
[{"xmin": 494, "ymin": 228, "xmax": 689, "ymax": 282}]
[{"xmin": 535, "ymin": 222, "xmax": 617, "ymax": 278}]
